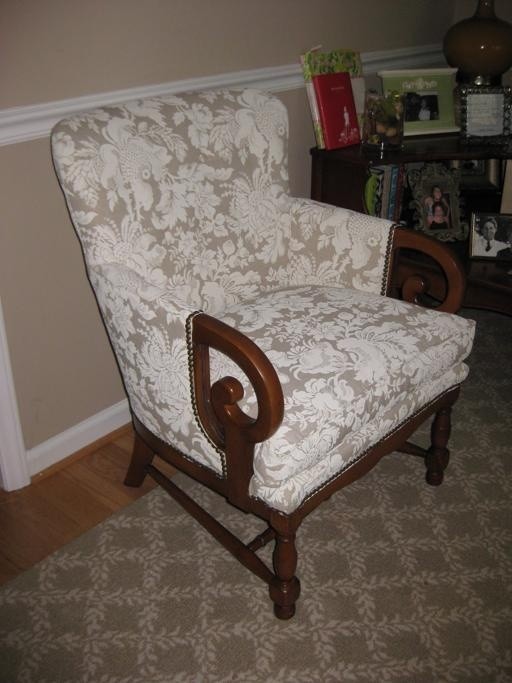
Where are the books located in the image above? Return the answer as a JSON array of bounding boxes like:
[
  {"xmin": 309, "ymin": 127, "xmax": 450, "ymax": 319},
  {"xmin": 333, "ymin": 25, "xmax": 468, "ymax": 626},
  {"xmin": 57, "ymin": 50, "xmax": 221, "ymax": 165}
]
[{"xmin": 300, "ymin": 43, "xmax": 367, "ymax": 150}]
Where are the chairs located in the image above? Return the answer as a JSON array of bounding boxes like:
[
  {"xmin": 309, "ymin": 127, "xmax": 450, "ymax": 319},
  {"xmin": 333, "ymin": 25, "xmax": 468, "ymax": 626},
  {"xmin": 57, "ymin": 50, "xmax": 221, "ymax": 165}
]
[{"xmin": 49, "ymin": 88, "xmax": 476, "ymax": 620}]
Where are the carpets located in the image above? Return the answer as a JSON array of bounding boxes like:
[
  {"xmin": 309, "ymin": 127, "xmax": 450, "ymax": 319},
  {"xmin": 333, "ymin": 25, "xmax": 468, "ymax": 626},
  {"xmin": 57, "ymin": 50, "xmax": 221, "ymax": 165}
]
[{"xmin": 0, "ymin": 300, "xmax": 511, "ymax": 683}]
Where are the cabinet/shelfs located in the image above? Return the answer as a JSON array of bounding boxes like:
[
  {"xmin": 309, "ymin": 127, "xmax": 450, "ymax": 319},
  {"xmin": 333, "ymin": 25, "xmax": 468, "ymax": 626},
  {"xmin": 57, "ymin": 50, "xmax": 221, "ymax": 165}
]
[{"xmin": 309, "ymin": 132, "xmax": 512, "ymax": 253}]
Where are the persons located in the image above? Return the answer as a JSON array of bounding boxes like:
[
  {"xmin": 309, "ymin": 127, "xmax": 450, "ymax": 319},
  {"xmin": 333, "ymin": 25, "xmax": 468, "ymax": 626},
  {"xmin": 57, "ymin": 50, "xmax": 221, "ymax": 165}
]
[
  {"xmin": 429, "ymin": 203, "xmax": 447, "ymax": 229},
  {"xmin": 404, "ymin": 94, "xmax": 423, "ymax": 120},
  {"xmin": 424, "ymin": 184, "xmax": 454, "ymax": 231},
  {"xmin": 472, "ymin": 215, "xmax": 510, "ymax": 257},
  {"xmin": 421, "ymin": 100, "xmax": 434, "ymax": 122}
]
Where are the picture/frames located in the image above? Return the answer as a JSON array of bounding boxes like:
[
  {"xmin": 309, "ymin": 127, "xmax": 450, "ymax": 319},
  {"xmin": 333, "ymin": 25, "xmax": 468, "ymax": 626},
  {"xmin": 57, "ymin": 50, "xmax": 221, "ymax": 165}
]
[
  {"xmin": 375, "ymin": 66, "xmax": 461, "ymax": 137},
  {"xmin": 456, "ymin": 82, "xmax": 511, "ymax": 149},
  {"xmin": 403, "ymin": 159, "xmax": 511, "ymax": 265}
]
